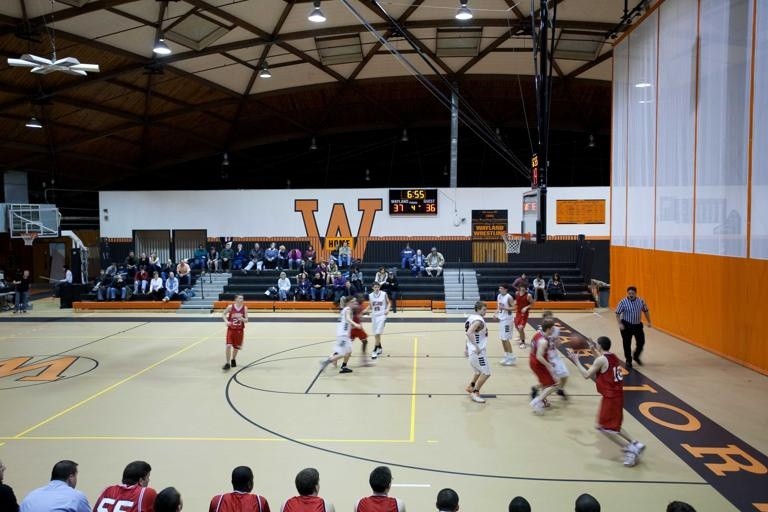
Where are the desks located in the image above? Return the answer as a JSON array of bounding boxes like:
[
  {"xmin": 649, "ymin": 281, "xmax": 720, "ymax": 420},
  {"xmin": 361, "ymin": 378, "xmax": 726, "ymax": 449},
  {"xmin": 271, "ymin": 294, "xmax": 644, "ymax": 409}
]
[{"xmin": 0, "ymin": 291, "xmax": 21, "ymax": 313}]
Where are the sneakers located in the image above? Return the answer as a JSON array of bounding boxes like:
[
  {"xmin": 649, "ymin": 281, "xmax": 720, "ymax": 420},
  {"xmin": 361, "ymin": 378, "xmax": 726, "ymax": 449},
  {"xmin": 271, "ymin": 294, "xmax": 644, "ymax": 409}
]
[
  {"xmin": 202, "ymin": 268, "xmax": 261, "ymax": 276},
  {"xmin": 393, "ymin": 310, "xmax": 396, "ymax": 313},
  {"xmin": 163, "ymin": 299, "xmax": 167, "ymax": 302}
]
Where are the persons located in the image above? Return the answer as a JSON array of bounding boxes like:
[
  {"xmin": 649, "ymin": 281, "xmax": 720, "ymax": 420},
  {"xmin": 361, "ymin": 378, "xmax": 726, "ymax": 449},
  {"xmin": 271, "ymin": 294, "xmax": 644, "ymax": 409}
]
[
  {"xmin": 574, "ymin": 493, "xmax": 601, "ymax": 512},
  {"xmin": 435, "ymin": 487, "xmax": 460, "ymax": 511},
  {"xmin": 194, "ymin": 243, "xmax": 264, "ymax": 275},
  {"xmin": 352, "ymin": 466, "xmax": 407, "ymax": 512},
  {"xmin": 508, "ymin": 495, "xmax": 531, "ymax": 512},
  {"xmin": 265, "ymin": 242, "xmax": 363, "ymax": 311},
  {"xmin": 154, "ymin": 486, "xmax": 183, "ymax": 512},
  {"xmin": 464, "ymin": 301, "xmax": 492, "ymax": 403},
  {"xmin": 359, "ymin": 280, "xmax": 392, "ymax": 360},
  {"xmin": 279, "ymin": 467, "xmax": 337, "ymax": 512},
  {"xmin": 92, "ymin": 460, "xmax": 158, "ymax": 512},
  {"xmin": 319, "ymin": 296, "xmax": 362, "ymax": 375},
  {"xmin": 400, "ymin": 243, "xmax": 445, "ymax": 277},
  {"xmin": 666, "ymin": 501, "xmax": 698, "ymax": 512},
  {"xmin": 92, "ymin": 251, "xmax": 191, "ymax": 302},
  {"xmin": 19, "ymin": 458, "xmax": 93, "ymax": 512},
  {"xmin": 0, "ymin": 460, "xmax": 20, "ymax": 512},
  {"xmin": 496, "ymin": 271, "xmax": 652, "ymax": 469},
  {"xmin": 207, "ymin": 466, "xmax": 272, "ymax": 512},
  {"xmin": 50, "ymin": 263, "xmax": 73, "ymax": 285},
  {"xmin": 11, "ymin": 268, "xmax": 31, "ymax": 315},
  {"xmin": 220, "ymin": 294, "xmax": 248, "ymax": 370},
  {"xmin": 375, "ymin": 268, "xmax": 399, "ymax": 299},
  {"xmin": 328, "ymin": 292, "xmax": 377, "ymax": 370}
]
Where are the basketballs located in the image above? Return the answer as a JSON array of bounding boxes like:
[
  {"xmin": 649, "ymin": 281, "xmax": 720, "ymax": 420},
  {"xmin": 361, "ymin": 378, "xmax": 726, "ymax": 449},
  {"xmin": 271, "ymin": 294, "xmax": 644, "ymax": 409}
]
[{"xmin": 569, "ymin": 333, "xmax": 586, "ymax": 350}]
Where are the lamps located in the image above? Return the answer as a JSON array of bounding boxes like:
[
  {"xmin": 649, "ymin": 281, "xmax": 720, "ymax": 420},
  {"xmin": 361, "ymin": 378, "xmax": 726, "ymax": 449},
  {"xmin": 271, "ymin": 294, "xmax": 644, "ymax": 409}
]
[
  {"xmin": 454, "ymin": 1, "xmax": 473, "ymax": 20},
  {"xmin": 152, "ymin": 23, "xmax": 171, "ymax": 54},
  {"xmin": 259, "ymin": 62, "xmax": 271, "ymax": 78},
  {"xmin": 25, "ymin": 116, "xmax": 43, "ymax": 128},
  {"xmin": 307, "ymin": 1, "xmax": 327, "ymax": 22},
  {"xmin": 7, "ymin": 0, "xmax": 100, "ymax": 76}
]
